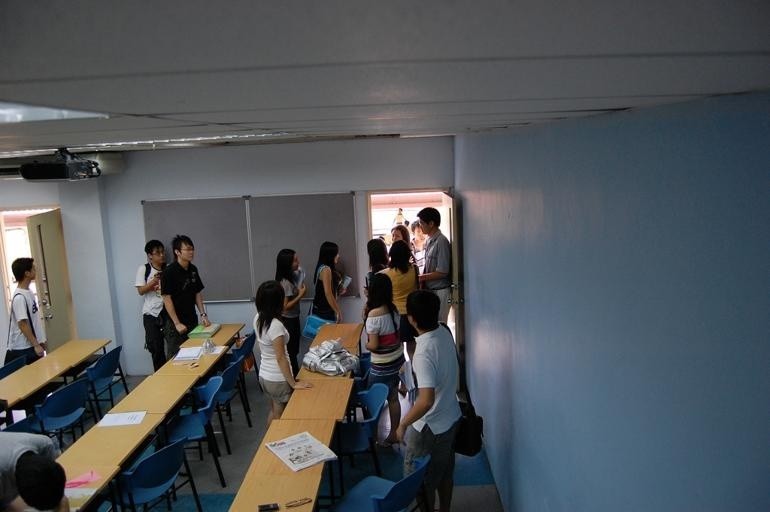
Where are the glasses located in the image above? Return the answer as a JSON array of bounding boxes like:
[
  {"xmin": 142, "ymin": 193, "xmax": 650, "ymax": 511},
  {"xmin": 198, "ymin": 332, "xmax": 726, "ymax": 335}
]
[
  {"xmin": 150, "ymin": 250, "xmax": 165, "ymax": 256},
  {"xmin": 180, "ymin": 247, "xmax": 195, "ymax": 251}
]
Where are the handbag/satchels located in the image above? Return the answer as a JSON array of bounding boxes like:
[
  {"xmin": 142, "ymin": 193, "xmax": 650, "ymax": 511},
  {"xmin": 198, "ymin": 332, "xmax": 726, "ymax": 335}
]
[
  {"xmin": 301, "ymin": 314, "xmax": 339, "ymax": 341},
  {"xmin": 448, "ymin": 400, "xmax": 484, "ymax": 457},
  {"xmin": 3, "ymin": 345, "xmax": 40, "ymax": 365}
]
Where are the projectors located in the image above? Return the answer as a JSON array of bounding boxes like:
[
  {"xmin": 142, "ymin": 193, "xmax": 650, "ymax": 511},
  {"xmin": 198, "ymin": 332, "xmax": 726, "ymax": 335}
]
[{"xmin": 20, "ymin": 159, "xmax": 92, "ymax": 178}]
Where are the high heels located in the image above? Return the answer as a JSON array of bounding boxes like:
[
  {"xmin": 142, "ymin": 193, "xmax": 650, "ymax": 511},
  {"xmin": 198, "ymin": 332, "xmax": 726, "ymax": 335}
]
[{"xmin": 376, "ymin": 438, "xmax": 402, "ymax": 451}]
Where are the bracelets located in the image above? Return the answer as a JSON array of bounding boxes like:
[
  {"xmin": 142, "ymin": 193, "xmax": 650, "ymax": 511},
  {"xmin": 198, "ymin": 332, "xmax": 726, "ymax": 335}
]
[
  {"xmin": 290, "ymin": 381, "xmax": 297, "ymax": 386},
  {"xmin": 201, "ymin": 313, "xmax": 208, "ymax": 317}
]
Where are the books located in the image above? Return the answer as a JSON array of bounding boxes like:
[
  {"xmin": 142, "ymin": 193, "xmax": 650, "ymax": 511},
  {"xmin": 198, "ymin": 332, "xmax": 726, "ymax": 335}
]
[
  {"xmin": 188, "ymin": 321, "xmax": 221, "ymax": 338},
  {"xmin": 265, "ymin": 431, "xmax": 339, "ymax": 472}
]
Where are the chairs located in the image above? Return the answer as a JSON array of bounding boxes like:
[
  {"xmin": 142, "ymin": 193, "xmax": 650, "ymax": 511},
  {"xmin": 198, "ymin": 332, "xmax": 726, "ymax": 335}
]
[{"xmin": 1, "ymin": 320, "xmax": 437, "ymax": 511}]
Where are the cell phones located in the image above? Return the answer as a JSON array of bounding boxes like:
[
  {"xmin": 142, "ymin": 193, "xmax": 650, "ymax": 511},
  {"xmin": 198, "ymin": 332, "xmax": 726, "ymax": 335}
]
[{"xmin": 258, "ymin": 504, "xmax": 278, "ymax": 512}]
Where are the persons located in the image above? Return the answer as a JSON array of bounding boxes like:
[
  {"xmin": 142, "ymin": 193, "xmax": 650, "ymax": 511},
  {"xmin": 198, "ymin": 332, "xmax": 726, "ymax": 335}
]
[
  {"xmin": 3, "ymin": 258, "xmax": 48, "ymax": 364},
  {"xmin": 396, "ymin": 289, "xmax": 462, "ymax": 511},
  {"xmin": 274, "ymin": 248, "xmax": 307, "ymax": 378},
  {"xmin": 358, "ymin": 207, "xmax": 454, "ymax": 398},
  {"xmin": 0, "ymin": 431, "xmax": 71, "ymax": 511},
  {"xmin": 161, "ymin": 233, "xmax": 210, "ymax": 362},
  {"xmin": 251, "ymin": 280, "xmax": 312, "ymax": 431},
  {"xmin": 307, "ymin": 241, "xmax": 348, "ymax": 348},
  {"xmin": 361, "ymin": 273, "xmax": 405, "ymax": 451},
  {"xmin": 134, "ymin": 239, "xmax": 180, "ymax": 374}
]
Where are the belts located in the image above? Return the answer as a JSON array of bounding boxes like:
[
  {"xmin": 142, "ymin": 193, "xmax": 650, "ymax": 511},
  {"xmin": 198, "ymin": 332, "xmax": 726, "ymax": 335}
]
[{"xmin": 430, "ymin": 284, "xmax": 451, "ymax": 290}]
